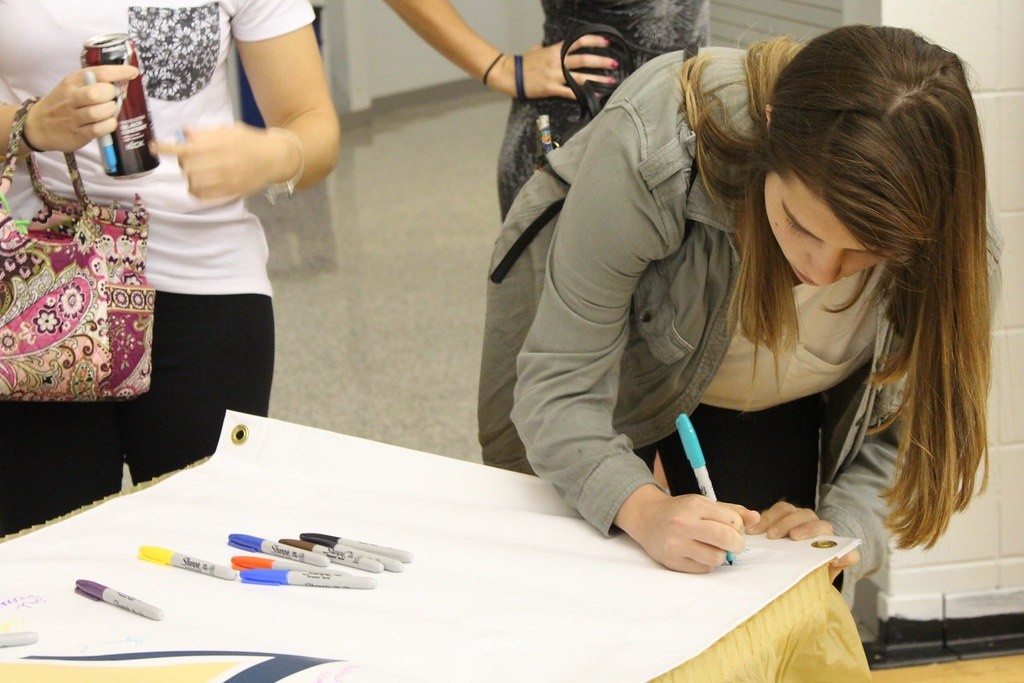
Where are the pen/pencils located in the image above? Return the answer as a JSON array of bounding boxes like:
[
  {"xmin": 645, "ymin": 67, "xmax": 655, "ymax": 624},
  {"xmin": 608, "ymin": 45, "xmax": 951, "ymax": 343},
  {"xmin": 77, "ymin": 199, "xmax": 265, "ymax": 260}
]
[
  {"xmin": 277, "ymin": 537, "xmax": 384, "ymax": 574},
  {"xmin": 1, "ymin": 631, "xmax": 37, "ymax": 647},
  {"xmin": 74, "ymin": 579, "xmax": 165, "ymax": 621},
  {"xmin": 299, "ymin": 532, "xmax": 414, "ymax": 564},
  {"xmin": 138, "ymin": 545, "xmax": 237, "ymax": 579},
  {"xmin": 230, "ymin": 556, "xmax": 335, "ymax": 573},
  {"xmin": 239, "ymin": 568, "xmax": 379, "ymax": 589},
  {"xmin": 84, "ymin": 71, "xmax": 117, "ymax": 174},
  {"xmin": 227, "ymin": 533, "xmax": 331, "ymax": 567},
  {"xmin": 675, "ymin": 412, "xmax": 732, "ymax": 564},
  {"xmin": 306, "ymin": 536, "xmax": 405, "ymax": 572}
]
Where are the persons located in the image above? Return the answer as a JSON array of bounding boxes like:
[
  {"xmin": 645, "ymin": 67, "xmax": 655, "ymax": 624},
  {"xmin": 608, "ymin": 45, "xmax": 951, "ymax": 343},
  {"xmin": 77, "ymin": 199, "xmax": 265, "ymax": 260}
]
[
  {"xmin": 477, "ymin": 24, "xmax": 1003, "ymax": 609},
  {"xmin": 0, "ymin": 0, "xmax": 342, "ymax": 535},
  {"xmin": 383, "ymin": 0, "xmax": 711, "ymax": 221}
]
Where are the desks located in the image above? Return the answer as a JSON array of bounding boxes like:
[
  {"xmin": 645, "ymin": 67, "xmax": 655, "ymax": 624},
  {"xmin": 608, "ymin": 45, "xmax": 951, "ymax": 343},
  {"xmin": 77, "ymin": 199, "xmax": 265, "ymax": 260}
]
[{"xmin": 0, "ymin": 454, "xmax": 873, "ymax": 683}]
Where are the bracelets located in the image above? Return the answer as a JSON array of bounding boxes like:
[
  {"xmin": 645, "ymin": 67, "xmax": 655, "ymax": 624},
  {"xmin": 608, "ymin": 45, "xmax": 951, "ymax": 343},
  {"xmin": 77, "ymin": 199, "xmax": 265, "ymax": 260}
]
[
  {"xmin": 483, "ymin": 53, "xmax": 503, "ymax": 85},
  {"xmin": 515, "ymin": 55, "xmax": 529, "ymax": 100},
  {"xmin": 265, "ymin": 126, "xmax": 305, "ymax": 206},
  {"xmin": 21, "ymin": 125, "xmax": 45, "ymax": 153}
]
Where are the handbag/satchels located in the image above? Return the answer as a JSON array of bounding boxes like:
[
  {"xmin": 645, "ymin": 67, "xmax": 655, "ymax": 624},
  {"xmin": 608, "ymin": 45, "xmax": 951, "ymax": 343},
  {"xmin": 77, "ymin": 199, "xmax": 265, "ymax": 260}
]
[{"xmin": 0, "ymin": 99, "xmax": 156, "ymax": 402}]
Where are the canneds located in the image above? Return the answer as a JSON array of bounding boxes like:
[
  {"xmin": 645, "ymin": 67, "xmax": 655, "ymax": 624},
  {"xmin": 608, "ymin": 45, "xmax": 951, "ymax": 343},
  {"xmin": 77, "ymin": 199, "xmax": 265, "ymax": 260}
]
[{"xmin": 80, "ymin": 32, "xmax": 162, "ymax": 180}]
